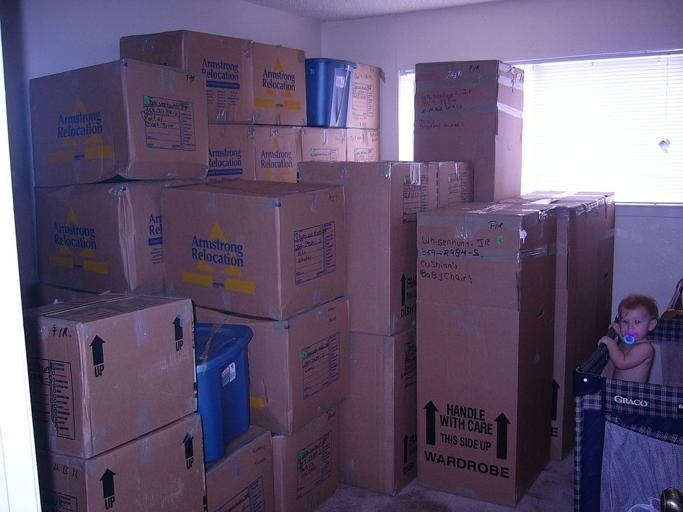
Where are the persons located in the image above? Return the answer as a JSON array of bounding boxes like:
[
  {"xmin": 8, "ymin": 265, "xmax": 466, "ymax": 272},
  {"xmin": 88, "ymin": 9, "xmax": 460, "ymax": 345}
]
[{"xmin": 597, "ymin": 294, "xmax": 659, "ymax": 384}]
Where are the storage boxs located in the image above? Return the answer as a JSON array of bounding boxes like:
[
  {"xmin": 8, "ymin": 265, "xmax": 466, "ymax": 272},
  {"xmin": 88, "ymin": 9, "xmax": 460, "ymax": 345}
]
[
  {"xmin": 296, "ymin": 158, "xmax": 474, "ymax": 336},
  {"xmin": 411, "ymin": 58, "xmax": 526, "ymax": 201},
  {"xmin": 36, "ymin": 412, "xmax": 208, "ymax": 512},
  {"xmin": 499, "ymin": 189, "xmax": 614, "ymax": 460},
  {"xmin": 273, "ymin": 404, "xmax": 343, "ymax": 512},
  {"xmin": 206, "ymin": 425, "xmax": 275, "ymax": 512},
  {"xmin": 30, "ymin": 180, "xmax": 210, "ymax": 292},
  {"xmin": 193, "ymin": 296, "xmax": 350, "ymax": 436},
  {"xmin": 160, "ymin": 178, "xmax": 349, "ymax": 321},
  {"xmin": 26, "ymin": 55, "xmax": 210, "ymax": 189},
  {"xmin": 23, "ymin": 291, "xmax": 198, "ymax": 460},
  {"xmin": 415, "ymin": 202, "xmax": 558, "ymax": 507},
  {"xmin": 194, "ymin": 321, "xmax": 254, "ymax": 463},
  {"xmin": 117, "ymin": 30, "xmax": 386, "ymax": 184},
  {"xmin": 347, "ymin": 329, "xmax": 415, "ymax": 498}
]
